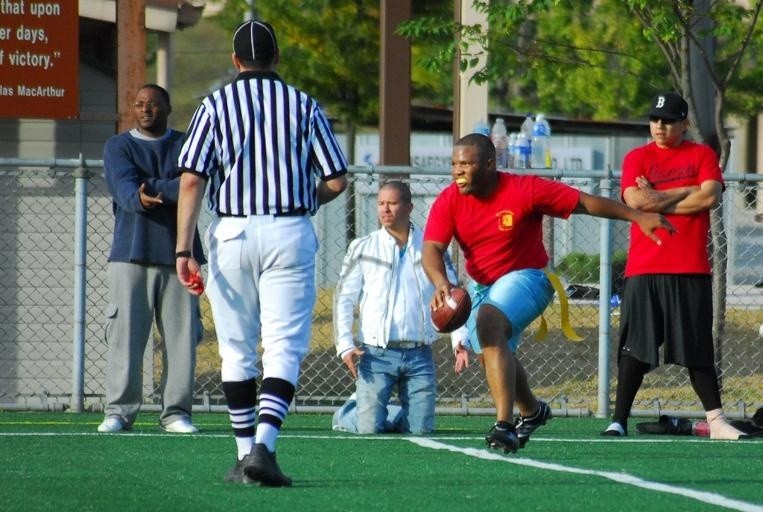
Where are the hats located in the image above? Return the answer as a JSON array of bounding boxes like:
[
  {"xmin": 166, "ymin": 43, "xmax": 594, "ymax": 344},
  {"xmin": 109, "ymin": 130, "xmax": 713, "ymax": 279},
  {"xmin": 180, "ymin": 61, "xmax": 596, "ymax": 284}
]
[
  {"xmin": 233, "ymin": 19, "xmax": 277, "ymax": 61},
  {"xmin": 645, "ymin": 92, "xmax": 689, "ymax": 119}
]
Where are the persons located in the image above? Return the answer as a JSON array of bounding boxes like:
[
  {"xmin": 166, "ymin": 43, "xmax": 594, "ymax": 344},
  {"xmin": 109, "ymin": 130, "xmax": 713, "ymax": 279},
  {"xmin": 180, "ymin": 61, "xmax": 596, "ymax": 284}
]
[
  {"xmin": 95, "ymin": 85, "xmax": 207, "ymax": 435},
  {"xmin": 331, "ymin": 182, "xmax": 469, "ymax": 435},
  {"xmin": 421, "ymin": 133, "xmax": 678, "ymax": 454},
  {"xmin": 174, "ymin": 18, "xmax": 349, "ymax": 486},
  {"xmin": 600, "ymin": 93, "xmax": 751, "ymax": 441}
]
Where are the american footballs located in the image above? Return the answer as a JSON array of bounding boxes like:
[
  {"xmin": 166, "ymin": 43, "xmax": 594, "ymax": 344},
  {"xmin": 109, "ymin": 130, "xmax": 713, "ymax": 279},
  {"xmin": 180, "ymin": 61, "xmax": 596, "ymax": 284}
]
[{"xmin": 430, "ymin": 287, "xmax": 471, "ymax": 333}]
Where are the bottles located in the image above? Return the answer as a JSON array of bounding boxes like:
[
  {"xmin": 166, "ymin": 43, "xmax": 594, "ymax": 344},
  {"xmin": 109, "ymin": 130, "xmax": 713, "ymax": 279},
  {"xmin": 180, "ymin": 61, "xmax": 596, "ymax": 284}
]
[
  {"xmin": 610, "ymin": 294, "xmax": 619, "ymax": 316},
  {"xmin": 478, "ymin": 110, "xmax": 555, "ymax": 168},
  {"xmin": 690, "ymin": 421, "xmax": 711, "ymax": 436}
]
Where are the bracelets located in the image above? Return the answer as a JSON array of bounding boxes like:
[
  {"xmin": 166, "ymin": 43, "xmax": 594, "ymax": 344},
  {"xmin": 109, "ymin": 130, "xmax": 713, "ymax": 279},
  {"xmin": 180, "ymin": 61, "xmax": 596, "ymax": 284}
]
[{"xmin": 174, "ymin": 251, "xmax": 193, "ymax": 259}]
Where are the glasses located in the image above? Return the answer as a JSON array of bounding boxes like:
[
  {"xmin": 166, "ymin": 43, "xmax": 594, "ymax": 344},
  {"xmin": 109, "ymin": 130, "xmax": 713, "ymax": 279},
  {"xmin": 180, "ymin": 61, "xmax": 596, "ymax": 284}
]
[
  {"xmin": 648, "ymin": 115, "xmax": 676, "ymax": 125},
  {"xmin": 129, "ymin": 100, "xmax": 166, "ymax": 110}
]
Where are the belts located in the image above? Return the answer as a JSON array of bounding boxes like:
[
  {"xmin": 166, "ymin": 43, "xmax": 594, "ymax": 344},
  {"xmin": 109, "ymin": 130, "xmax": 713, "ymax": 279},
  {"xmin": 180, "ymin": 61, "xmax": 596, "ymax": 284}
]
[
  {"xmin": 216, "ymin": 209, "xmax": 307, "ymax": 217},
  {"xmin": 388, "ymin": 340, "xmax": 425, "ymax": 349}
]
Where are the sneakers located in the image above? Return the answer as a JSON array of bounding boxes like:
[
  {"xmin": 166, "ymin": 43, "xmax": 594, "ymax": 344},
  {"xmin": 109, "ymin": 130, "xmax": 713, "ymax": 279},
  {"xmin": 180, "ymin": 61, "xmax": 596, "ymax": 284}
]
[
  {"xmin": 485, "ymin": 421, "xmax": 520, "ymax": 455},
  {"xmin": 515, "ymin": 400, "xmax": 552, "ymax": 448},
  {"xmin": 331, "ymin": 393, "xmax": 356, "ymax": 432},
  {"xmin": 98, "ymin": 417, "xmax": 124, "ymax": 432},
  {"xmin": 160, "ymin": 417, "xmax": 200, "ymax": 434},
  {"xmin": 224, "ymin": 443, "xmax": 293, "ymax": 487}
]
